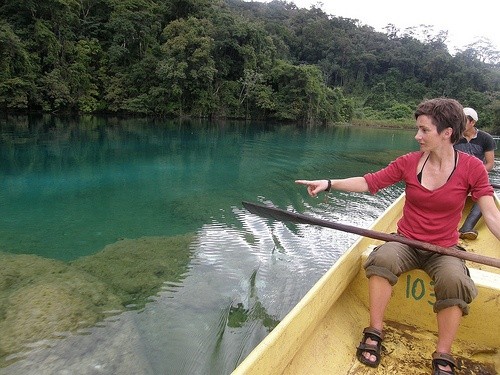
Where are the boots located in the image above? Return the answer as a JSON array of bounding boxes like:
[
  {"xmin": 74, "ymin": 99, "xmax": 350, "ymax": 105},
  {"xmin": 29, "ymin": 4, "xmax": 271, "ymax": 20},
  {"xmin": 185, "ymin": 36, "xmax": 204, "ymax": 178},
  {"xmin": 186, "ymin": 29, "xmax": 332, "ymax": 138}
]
[{"xmin": 458, "ymin": 200, "xmax": 483, "ymax": 240}]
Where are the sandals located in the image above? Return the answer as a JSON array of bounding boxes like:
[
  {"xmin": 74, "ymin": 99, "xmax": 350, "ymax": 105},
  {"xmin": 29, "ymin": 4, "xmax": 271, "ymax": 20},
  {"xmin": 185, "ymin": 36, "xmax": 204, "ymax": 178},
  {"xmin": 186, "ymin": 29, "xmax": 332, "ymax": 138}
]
[
  {"xmin": 431, "ymin": 351, "xmax": 457, "ymax": 375},
  {"xmin": 356, "ymin": 326, "xmax": 384, "ymax": 368}
]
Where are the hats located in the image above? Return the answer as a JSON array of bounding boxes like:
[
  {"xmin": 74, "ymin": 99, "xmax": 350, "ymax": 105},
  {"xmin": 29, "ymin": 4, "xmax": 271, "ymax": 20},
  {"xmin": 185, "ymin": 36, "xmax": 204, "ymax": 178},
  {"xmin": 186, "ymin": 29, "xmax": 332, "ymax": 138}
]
[{"xmin": 463, "ymin": 107, "xmax": 479, "ymax": 123}]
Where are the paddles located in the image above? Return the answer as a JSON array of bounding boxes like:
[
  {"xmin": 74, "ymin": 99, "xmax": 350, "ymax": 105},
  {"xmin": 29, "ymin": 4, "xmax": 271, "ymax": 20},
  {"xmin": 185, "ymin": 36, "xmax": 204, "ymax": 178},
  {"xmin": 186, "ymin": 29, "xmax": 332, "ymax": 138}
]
[{"xmin": 240, "ymin": 199, "xmax": 500, "ymax": 269}]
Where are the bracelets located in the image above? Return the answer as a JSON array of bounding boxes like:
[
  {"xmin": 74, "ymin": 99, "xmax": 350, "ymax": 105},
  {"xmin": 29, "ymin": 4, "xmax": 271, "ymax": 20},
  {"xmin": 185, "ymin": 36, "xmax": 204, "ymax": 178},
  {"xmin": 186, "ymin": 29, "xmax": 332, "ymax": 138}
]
[{"xmin": 323, "ymin": 178, "xmax": 332, "ymax": 192}]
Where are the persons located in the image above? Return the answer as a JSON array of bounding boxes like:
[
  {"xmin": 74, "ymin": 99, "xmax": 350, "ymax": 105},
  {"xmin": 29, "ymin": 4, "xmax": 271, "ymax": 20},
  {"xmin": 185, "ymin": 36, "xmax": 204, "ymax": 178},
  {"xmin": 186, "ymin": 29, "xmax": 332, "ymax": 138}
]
[
  {"xmin": 295, "ymin": 98, "xmax": 500, "ymax": 375},
  {"xmin": 453, "ymin": 107, "xmax": 498, "ymax": 241}
]
